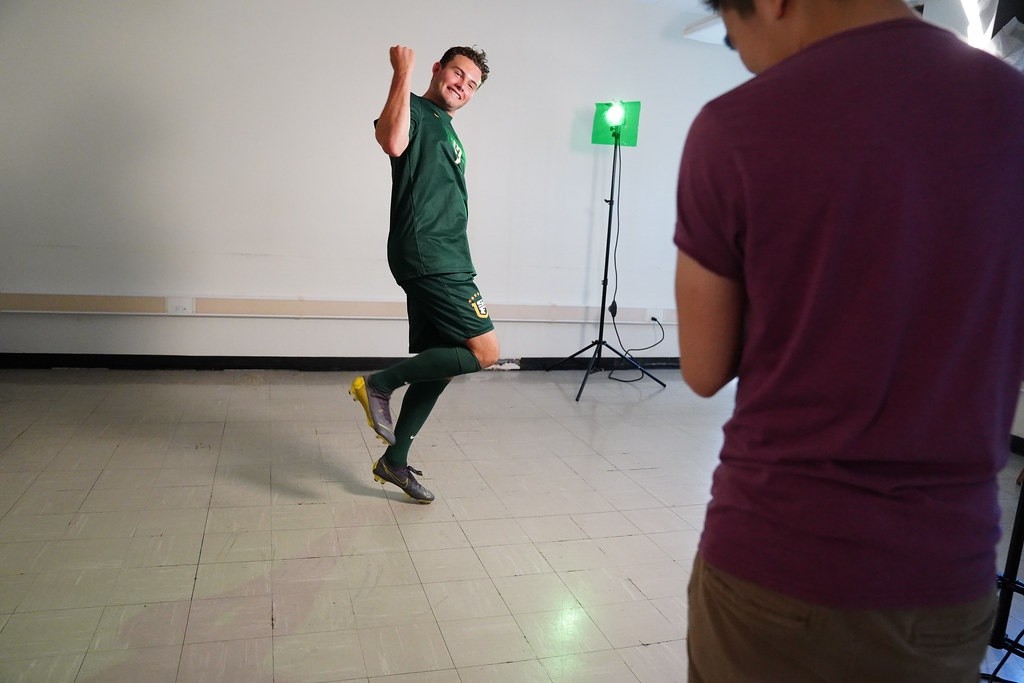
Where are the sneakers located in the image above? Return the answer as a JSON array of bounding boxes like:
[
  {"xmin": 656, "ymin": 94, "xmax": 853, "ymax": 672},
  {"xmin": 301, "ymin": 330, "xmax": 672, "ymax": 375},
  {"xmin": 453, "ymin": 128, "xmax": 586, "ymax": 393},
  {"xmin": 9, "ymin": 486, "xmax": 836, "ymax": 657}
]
[
  {"xmin": 373, "ymin": 456, "xmax": 435, "ymax": 505},
  {"xmin": 347, "ymin": 374, "xmax": 396, "ymax": 445}
]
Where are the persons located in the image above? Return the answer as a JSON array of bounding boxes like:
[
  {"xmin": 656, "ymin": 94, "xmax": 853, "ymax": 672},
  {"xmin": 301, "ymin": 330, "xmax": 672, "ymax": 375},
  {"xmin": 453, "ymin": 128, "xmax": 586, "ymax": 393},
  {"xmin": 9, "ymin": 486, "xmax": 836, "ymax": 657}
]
[
  {"xmin": 672, "ymin": 0, "xmax": 1024, "ymax": 683},
  {"xmin": 345, "ymin": 44, "xmax": 500, "ymax": 503}
]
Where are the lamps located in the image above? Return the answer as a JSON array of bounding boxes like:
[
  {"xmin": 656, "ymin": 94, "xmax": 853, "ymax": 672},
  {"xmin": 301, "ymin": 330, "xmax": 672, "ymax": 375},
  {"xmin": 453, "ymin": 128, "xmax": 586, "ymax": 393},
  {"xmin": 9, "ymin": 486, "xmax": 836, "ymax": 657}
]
[{"xmin": 605, "ymin": 103, "xmax": 627, "ymax": 128}]
